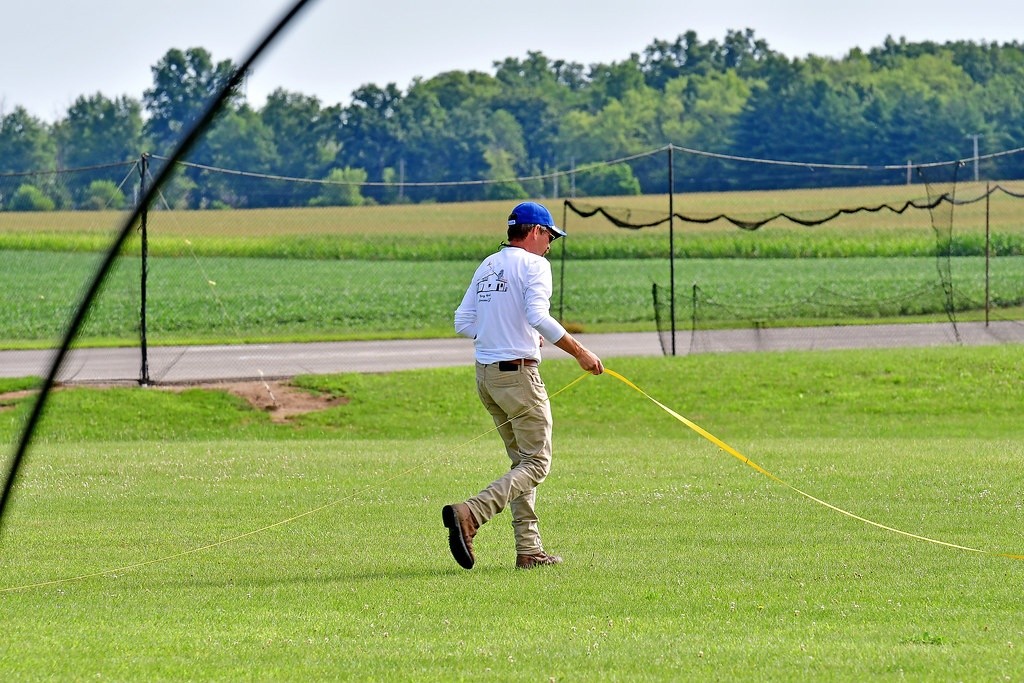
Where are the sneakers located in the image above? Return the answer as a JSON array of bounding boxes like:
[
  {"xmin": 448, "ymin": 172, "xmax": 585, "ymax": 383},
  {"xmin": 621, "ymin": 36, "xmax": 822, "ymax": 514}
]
[
  {"xmin": 515, "ymin": 551, "xmax": 562, "ymax": 569},
  {"xmin": 442, "ymin": 503, "xmax": 480, "ymax": 569}
]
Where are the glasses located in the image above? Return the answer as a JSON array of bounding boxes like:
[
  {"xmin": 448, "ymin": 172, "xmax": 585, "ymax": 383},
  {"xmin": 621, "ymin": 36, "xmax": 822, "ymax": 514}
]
[{"xmin": 532, "ymin": 224, "xmax": 555, "ymax": 244}]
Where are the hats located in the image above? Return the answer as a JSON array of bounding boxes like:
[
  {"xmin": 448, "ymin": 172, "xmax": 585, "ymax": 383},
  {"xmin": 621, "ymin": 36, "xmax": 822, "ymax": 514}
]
[{"xmin": 508, "ymin": 202, "xmax": 568, "ymax": 240}]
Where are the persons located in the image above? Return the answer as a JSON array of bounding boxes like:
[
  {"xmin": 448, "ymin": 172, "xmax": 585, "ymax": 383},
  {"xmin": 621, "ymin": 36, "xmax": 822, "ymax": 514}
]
[{"xmin": 442, "ymin": 203, "xmax": 603, "ymax": 571}]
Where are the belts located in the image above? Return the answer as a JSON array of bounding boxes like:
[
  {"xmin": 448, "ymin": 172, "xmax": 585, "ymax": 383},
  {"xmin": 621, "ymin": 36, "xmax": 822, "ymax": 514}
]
[{"xmin": 509, "ymin": 358, "xmax": 537, "ymax": 368}]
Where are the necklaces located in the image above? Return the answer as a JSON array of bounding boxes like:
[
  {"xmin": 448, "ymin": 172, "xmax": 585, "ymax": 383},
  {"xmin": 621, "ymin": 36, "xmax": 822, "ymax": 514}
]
[{"xmin": 498, "ymin": 240, "xmax": 527, "ymax": 251}]
